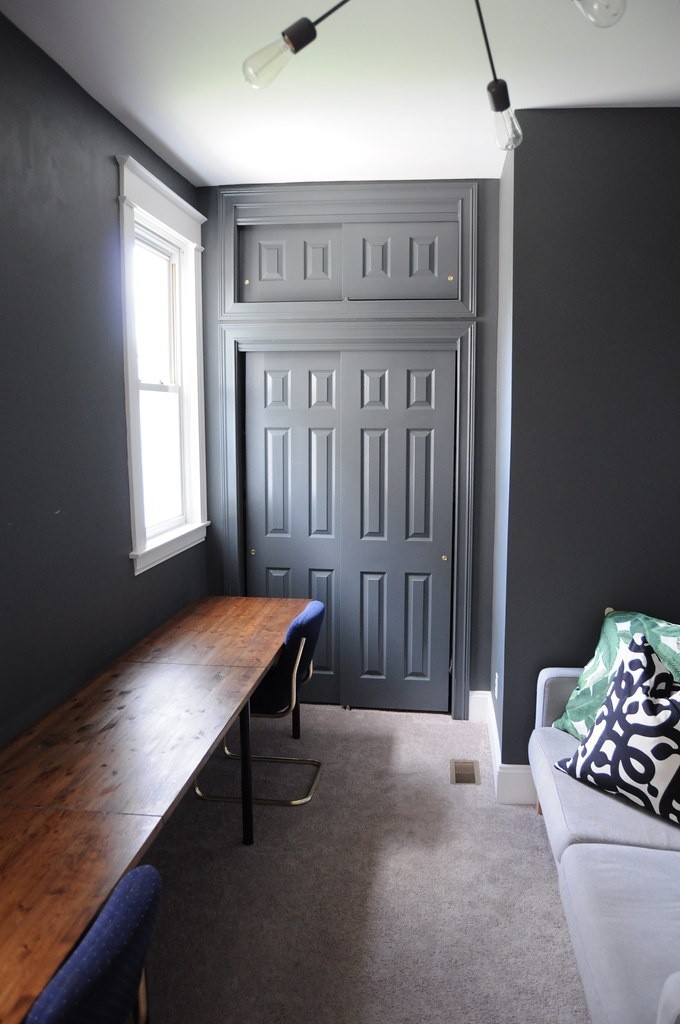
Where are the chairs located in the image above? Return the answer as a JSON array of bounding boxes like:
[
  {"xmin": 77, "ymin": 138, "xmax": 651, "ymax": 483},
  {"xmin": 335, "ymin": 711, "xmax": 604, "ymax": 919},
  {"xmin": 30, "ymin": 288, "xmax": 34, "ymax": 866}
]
[
  {"xmin": 25, "ymin": 864, "xmax": 163, "ymax": 1024},
  {"xmin": 192, "ymin": 600, "xmax": 326, "ymax": 807}
]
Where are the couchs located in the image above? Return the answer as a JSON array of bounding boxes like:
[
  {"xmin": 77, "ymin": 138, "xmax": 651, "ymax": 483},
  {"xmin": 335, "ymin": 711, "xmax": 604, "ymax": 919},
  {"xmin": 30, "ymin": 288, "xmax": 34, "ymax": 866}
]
[{"xmin": 528, "ymin": 667, "xmax": 680, "ymax": 1024}]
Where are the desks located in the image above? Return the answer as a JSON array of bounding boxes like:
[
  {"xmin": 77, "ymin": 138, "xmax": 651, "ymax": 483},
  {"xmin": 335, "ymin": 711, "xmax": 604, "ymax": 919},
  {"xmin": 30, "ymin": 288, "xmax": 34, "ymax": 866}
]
[{"xmin": 0, "ymin": 594, "xmax": 313, "ymax": 1024}]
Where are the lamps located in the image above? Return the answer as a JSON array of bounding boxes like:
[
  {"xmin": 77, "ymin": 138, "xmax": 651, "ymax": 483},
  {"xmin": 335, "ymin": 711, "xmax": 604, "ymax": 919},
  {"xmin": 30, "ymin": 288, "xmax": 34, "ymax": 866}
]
[{"xmin": 241, "ymin": 0, "xmax": 628, "ymax": 150}]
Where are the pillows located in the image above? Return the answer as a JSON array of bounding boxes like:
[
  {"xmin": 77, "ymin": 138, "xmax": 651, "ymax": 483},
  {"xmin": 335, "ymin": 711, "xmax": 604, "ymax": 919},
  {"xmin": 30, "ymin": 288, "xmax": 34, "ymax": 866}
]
[
  {"xmin": 554, "ymin": 633, "xmax": 680, "ymax": 825},
  {"xmin": 551, "ymin": 607, "xmax": 680, "ymax": 741}
]
